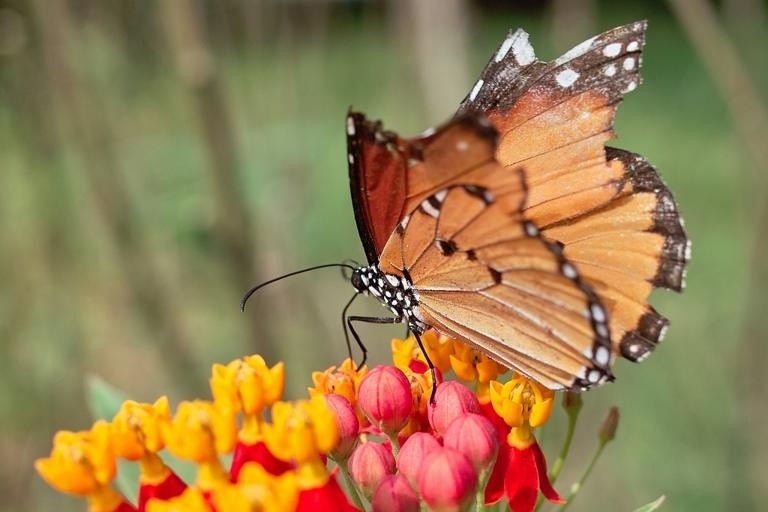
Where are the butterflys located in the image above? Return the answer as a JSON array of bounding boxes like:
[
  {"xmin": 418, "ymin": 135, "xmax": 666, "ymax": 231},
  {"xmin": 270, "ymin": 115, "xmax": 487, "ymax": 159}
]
[{"xmin": 238, "ymin": 16, "xmax": 693, "ymax": 410}]
